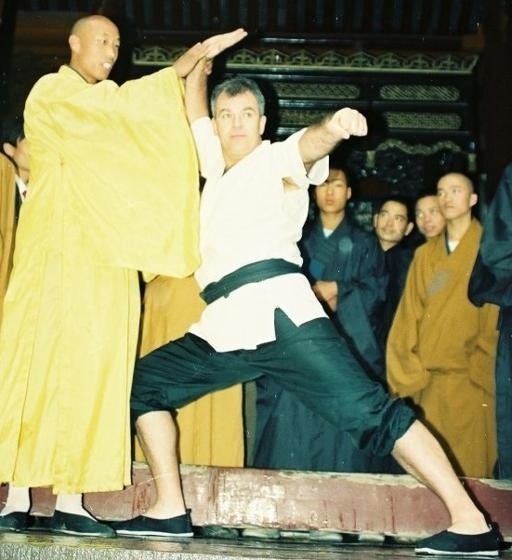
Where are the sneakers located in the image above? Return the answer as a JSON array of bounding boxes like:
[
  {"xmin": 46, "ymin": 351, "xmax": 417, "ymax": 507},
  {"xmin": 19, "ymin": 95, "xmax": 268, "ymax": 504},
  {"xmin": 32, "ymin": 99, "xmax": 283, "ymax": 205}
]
[
  {"xmin": 111, "ymin": 508, "xmax": 197, "ymax": 542},
  {"xmin": 1, "ymin": 511, "xmax": 28, "ymax": 532},
  {"xmin": 48, "ymin": 508, "xmax": 116, "ymax": 540},
  {"xmin": 410, "ymin": 523, "xmax": 501, "ymax": 559}
]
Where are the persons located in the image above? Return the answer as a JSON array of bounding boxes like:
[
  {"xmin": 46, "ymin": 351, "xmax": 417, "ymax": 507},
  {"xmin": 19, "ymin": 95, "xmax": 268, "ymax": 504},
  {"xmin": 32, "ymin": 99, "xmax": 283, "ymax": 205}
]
[
  {"xmin": 1, "ymin": 121, "xmax": 244, "ymax": 539},
  {"xmin": 115, "ymin": 28, "xmax": 506, "ymax": 559},
  {"xmin": 0, "ymin": 14, "xmax": 214, "ymax": 541},
  {"xmin": 241, "ymin": 161, "xmax": 509, "ymax": 543}
]
